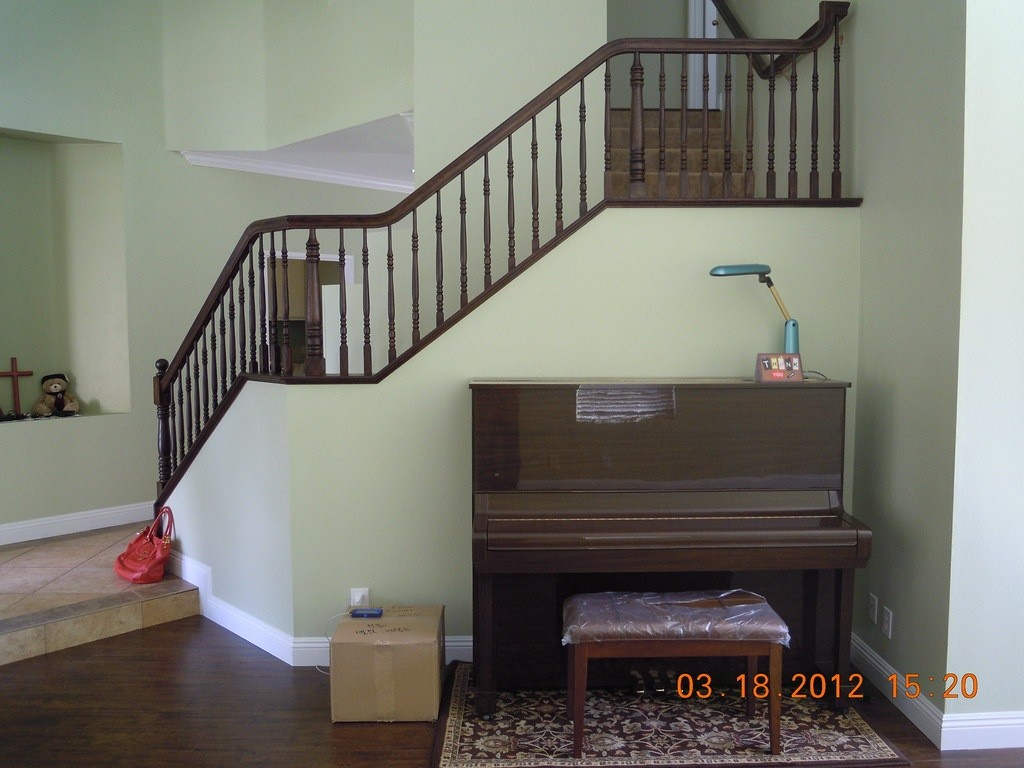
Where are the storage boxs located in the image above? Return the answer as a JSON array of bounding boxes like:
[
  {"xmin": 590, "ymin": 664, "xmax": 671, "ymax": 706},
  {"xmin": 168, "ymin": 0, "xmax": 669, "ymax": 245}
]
[{"xmin": 330, "ymin": 604, "xmax": 445, "ymax": 723}]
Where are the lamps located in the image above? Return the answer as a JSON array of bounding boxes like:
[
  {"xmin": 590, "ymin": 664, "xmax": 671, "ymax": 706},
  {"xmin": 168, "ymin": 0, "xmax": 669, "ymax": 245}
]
[{"xmin": 709, "ymin": 262, "xmax": 800, "ymax": 354}]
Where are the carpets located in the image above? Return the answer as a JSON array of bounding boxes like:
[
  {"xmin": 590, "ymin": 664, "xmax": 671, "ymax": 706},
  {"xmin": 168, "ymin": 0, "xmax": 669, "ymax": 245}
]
[{"xmin": 428, "ymin": 656, "xmax": 912, "ymax": 767}]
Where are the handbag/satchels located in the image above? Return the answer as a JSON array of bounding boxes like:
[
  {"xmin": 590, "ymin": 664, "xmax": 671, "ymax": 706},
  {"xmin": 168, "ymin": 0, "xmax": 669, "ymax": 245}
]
[{"xmin": 114, "ymin": 506, "xmax": 173, "ymax": 584}]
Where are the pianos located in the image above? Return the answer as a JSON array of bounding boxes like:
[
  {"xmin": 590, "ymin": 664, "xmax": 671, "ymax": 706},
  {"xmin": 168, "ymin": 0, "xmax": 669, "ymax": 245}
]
[{"xmin": 465, "ymin": 370, "xmax": 880, "ymax": 722}]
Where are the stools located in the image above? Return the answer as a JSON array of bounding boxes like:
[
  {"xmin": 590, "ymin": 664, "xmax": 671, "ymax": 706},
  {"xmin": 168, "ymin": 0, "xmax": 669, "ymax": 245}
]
[{"xmin": 561, "ymin": 588, "xmax": 791, "ymax": 759}]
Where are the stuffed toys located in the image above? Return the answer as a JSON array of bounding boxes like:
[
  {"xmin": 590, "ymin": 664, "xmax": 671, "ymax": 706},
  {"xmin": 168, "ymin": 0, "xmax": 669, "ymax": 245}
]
[{"xmin": 29, "ymin": 365, "xmax": 80, "ymax": 419}]
